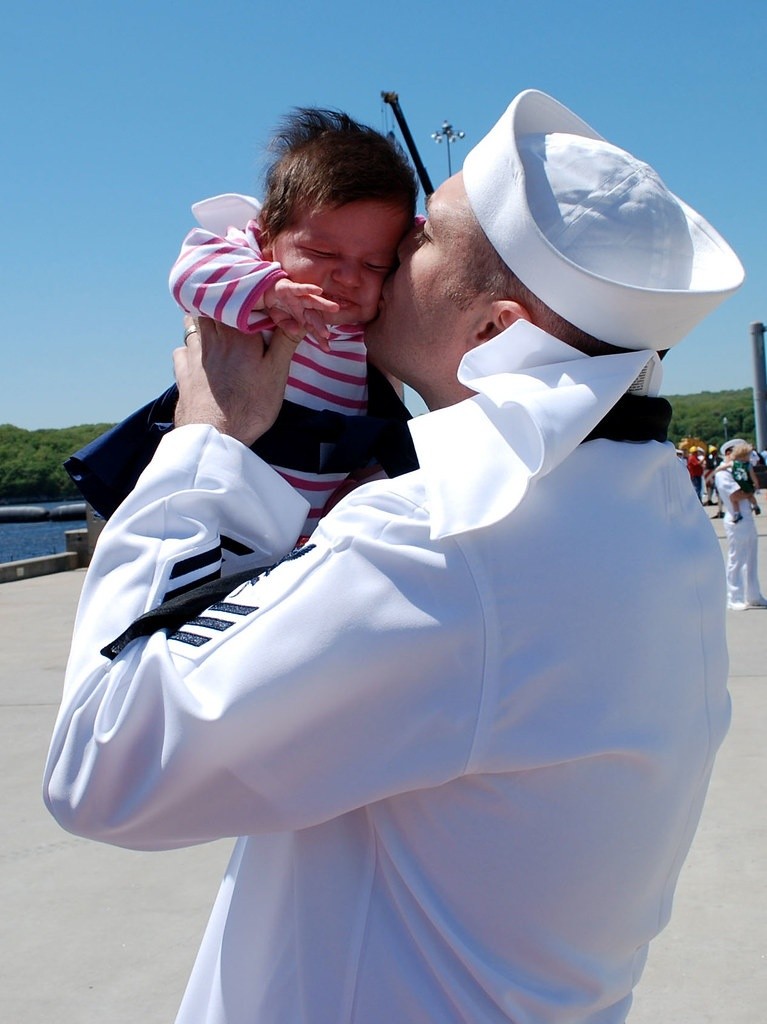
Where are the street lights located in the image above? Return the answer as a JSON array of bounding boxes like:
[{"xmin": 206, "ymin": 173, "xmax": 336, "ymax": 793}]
[{"xmin": 430, "ymin": 120, "xmax": 466, "ymax": 179}]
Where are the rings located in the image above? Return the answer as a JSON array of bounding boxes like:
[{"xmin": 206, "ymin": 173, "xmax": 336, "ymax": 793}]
[{"xmin": 183, "ymin": 324, "xmax": 198, "ymax": 347}]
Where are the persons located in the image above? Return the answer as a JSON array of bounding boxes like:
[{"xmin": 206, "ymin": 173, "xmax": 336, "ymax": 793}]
[
  {"xmin": 169, "ymin": 103, "xmax": 420, "ymax": 548},
  {"xmin": 714, "ymin": 439, "xmax": 767, "ymax": 612},
  {"xmin": 39, "ymin": 129, "xmax": 744, "ymax": 1024},
  {"xmin": 713, "ymin": 443, "xmax": 760, "ymax": 524},
  {"xmin": 675, "ymin": 438, "xmax": 767, "ymax": 520}
]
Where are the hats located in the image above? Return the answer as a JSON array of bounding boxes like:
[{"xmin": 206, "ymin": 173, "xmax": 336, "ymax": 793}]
[
  {"xmin": 462, "ymin": 89, "xmax": 744, "ymax": 352},
  {"xmin": 697, "ymin": 447, "xmax": 704, "ymax": 453},
  {"xmin": 720, "ymin": 439, "xmax": 746, "ymax": 455},
  {"xmin": 708, "ymin": 446, "xmax": 718, "ymax": 454},
  {"xmin": 676, "ymin": 450, "xmax": 683, "ymax": 457},
  {"xmin": 689, "ymin": 446, "xmax": 696, "ymax": 452}
]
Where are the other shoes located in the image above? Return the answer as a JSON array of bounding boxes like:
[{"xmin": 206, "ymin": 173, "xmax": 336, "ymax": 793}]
[
  {"xmin": 732, "ymin": 512, "xmax": 742, "ymax": 524},
  {"xmin": 726, "ymin": 601, "xmax": 747, "ymax": 611},
  {"xmin": 745, "ymin": 597, "xmax": 767, "ymax": 606},
  {"xmin": 753, "ymin": 506, "xmax": 760, "ymax": 515}
]
